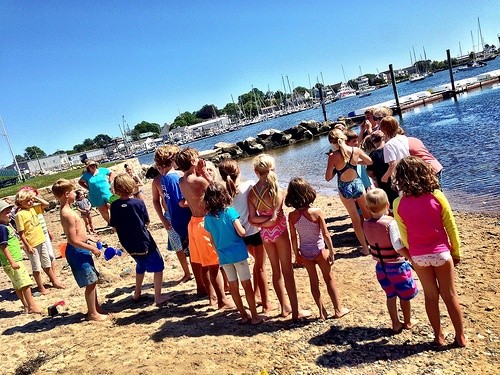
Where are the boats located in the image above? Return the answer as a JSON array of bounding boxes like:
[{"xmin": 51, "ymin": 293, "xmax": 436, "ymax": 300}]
[{"xmin": 358, "ymin": 92, "xmax": 371, "ymax": 99}]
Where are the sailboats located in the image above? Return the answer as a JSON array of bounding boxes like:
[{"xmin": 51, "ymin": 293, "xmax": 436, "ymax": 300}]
[
  {"xmin": 456, "ymin": 16, "xmax": 498, "ymax": 71},
  {"xmin": 178, "ymin": 74, "xmax": 389, "ymax": 146},
  {"xmin": 406, "ymin": 45, "xmax": 435, "ymax": 82}
]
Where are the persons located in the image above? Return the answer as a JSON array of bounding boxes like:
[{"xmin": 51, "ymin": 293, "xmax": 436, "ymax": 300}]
[
  {"xmin": 10, "ymin": 186, "xmax": 66, "ymax": 295},
  {"xmin": 52, "ymin": 179, "xmax": 111, "ymax": 321},
  {"xmin": 285, "ymin": 178, "xmax": 350, "ymax": 320},
  {"xmin": 203, "ymin": 182, "xmax": 267, "ymax": 325},
  {"xmin": 126, "ymin": 166, "xmax": 145, "ymax": 199},
  {"xmin": 110, "ymin": 174, "xmax": 172, "ymax": 306},
  {"xmin": 0, "ymin": 200, "xmax": 44, "ymax": 313},
  {"xmin": 76, "ymin": 190, "xmax": 96, "ymax": 235},
  {"xmin": 78, "ymin": 159, "xmax": 114, "ymax": 228},
  {"xmin": 392, "ymin": 156, "xmax": 468, "ymax": 347},
  {"xmin": 152, "ymin": 145, "xmax": 279, "ymax": 312},
  {"xmin": 326, "ymin": 107, "xmax": 443, "ymax": 256},
  {"xmin": 151, "ymin": 161, "xmax": 190, "ymax": 287},
  {"xmin": 203, "ymin": 159, "xmax": 217, "ymax": 181},
  {"xmin": 248, "ymin": 154, "xmax": 313, "ymax": 320},
  {"xmin": 361, "ymin": 188, "xmax": 419, "ymax": 332}
]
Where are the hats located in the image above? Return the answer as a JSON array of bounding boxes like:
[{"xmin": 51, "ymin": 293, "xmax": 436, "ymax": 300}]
[
  {"xmin": 0, "ymin": 198, "xmax": 15, "ymax": 214},
  {"xmin": 85, "ymin": 159, "xmax": 99, "ymax": 169},
  {"xmin": 19, "ymin": 186, "xmax": 39, "ymax": 195}
]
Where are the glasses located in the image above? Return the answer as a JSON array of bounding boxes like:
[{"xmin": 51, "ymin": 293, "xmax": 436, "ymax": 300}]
[{"xmin": 373, "ymin": 116, "xmax": 383, "ymax": 121}]
